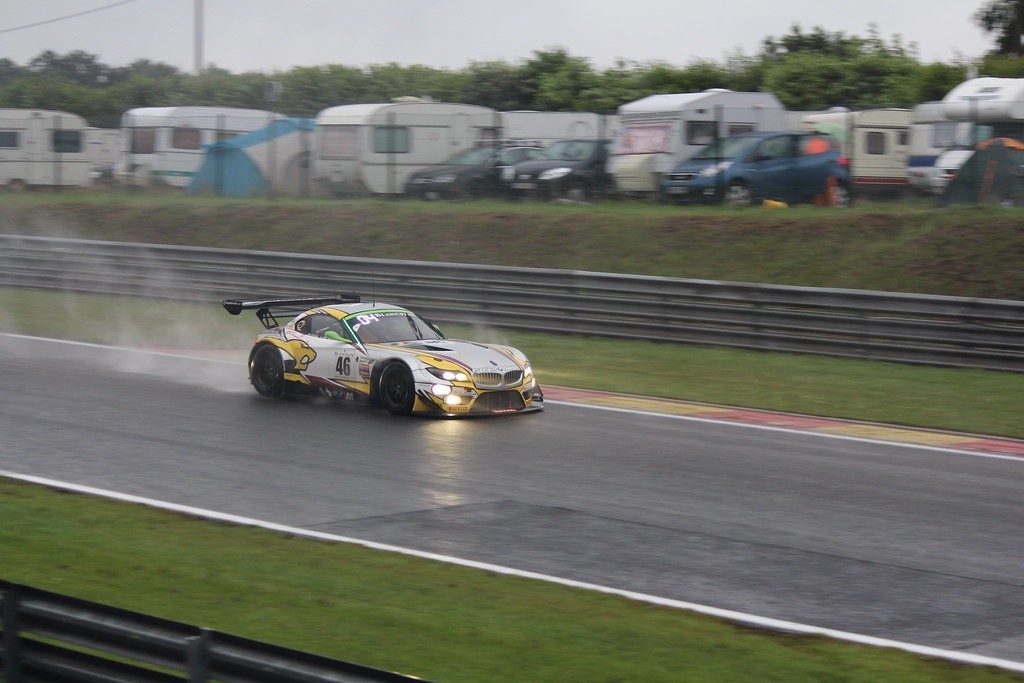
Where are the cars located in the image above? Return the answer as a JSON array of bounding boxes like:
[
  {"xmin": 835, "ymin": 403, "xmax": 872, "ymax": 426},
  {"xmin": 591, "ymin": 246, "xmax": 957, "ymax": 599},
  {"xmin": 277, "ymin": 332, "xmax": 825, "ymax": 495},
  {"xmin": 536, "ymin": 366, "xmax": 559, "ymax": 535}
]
[
  {"xmin": 900, "ymin": 148, "xmax": 978, "ymax": 198},
  {"xmin": 658, "ymin": 128, "xmax": 853, "ymax": 212},
  {"xmin": 404, "ymin": 143, "xmax": 542, "ymax": 205},
  {"xmin": 500, "ymin": 137, "xmax": 621, "ymax": 204}
]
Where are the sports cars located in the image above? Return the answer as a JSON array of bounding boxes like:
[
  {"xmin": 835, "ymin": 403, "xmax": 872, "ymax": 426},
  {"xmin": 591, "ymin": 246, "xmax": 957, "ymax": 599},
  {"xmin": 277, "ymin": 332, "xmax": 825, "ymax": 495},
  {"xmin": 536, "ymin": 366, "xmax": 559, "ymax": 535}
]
[{"xmin": 222, "ymin": 290, "xmax": 545, "ymax": 419}]
